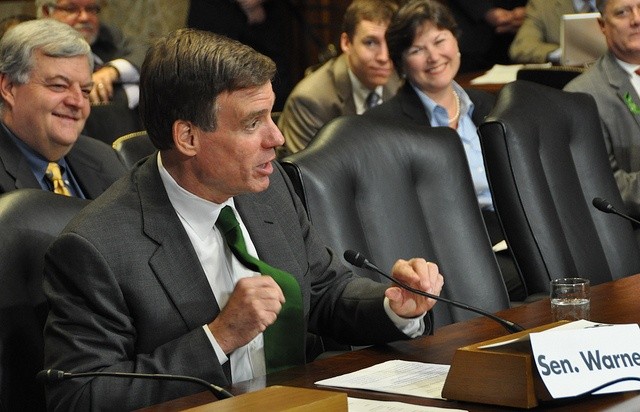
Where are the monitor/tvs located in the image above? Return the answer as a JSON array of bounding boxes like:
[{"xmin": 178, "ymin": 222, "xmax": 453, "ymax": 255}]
[{"xmin": 559, "ymin": 12, "xmax": 608, "ymax": 67}]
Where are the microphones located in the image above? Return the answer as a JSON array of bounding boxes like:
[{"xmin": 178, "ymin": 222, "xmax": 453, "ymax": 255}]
[
  {"xmin": 592, "ymin": 197, "xmax": 640, "ymax": 224},
  {"xmin": 344, "ymin": 250, "xmax": 526, "ymax": 334},
  {"xmin": 35, "ymin": 368, "xmax": 234, "ymax": 400}
]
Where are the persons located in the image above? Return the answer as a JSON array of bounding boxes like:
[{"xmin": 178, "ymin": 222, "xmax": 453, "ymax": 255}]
[
  {"xmin": 2, "ymin": 19, "xmax": 133, "ymax": 202},
  {"xmin": 190, "ymin": 1, "xmax": 284, "ymax": 65},
  {"xmin": 275, "ymin": 3, "xmax": 401, "ymax": 160},
  {"xmin": 40, "ymin": 29, "xmax": 446, "ymax": 407},
  {"xmin": 385, "ymin": 0, "xmax": 525, "ymax": 300},
  {"xmin": 451, "ymin": 2, "xmax": 528, "ymax": 64},
  {"xmin": 563, "ymin": 2, "xmax": 639, "ymax": 205},
  {"xmin": 34, "ymin": 0, "xmax": 145, "ymax": 104},
  {"xmin": 510, "ymin": 1, "xmax": 597, "ymax": 64}
]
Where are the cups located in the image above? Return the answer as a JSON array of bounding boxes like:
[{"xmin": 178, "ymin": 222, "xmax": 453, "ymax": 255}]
[{"xmin": 549, "ymin": 276, "xmax": 592, "ymax": 322}]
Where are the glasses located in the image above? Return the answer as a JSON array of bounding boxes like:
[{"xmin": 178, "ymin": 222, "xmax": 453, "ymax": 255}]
[{"xmin": 47, "ymin": 3, "xmax": 99, "ymax": 15}]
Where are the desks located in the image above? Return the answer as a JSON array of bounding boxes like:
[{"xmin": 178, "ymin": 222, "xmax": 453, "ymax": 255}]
[{"xmin": 127, "ymin": 269, "xmax": 640, "ymax": 412}]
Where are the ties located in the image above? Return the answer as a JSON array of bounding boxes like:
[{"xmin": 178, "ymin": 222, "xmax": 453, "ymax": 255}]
[
  {"xmin": 46, "ymin": 162, "xmax": 71, "ymax": 196},
  {"xmin": 366, "ymin": 93, "xmax": 380, "ymax": 106},
  {"xmin": 215, "ymin": 206, "xmax": 306, "ymax": 373}
]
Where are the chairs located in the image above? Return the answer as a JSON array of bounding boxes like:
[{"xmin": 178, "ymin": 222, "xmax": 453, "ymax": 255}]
[
  {"xmin": 476, "ymin": 80, "xmax": 639, "ymax": 301},
  {"xmin": 113, "ymin": 126, "xmax": 157, "ymax": 169},
  {"xmin": 0, "ymin": 187, "xmax": 125, "ymax": 412},
  {"xmin": 279, "ymin": 112, "xmax": 512, "ymax": 349}
]
